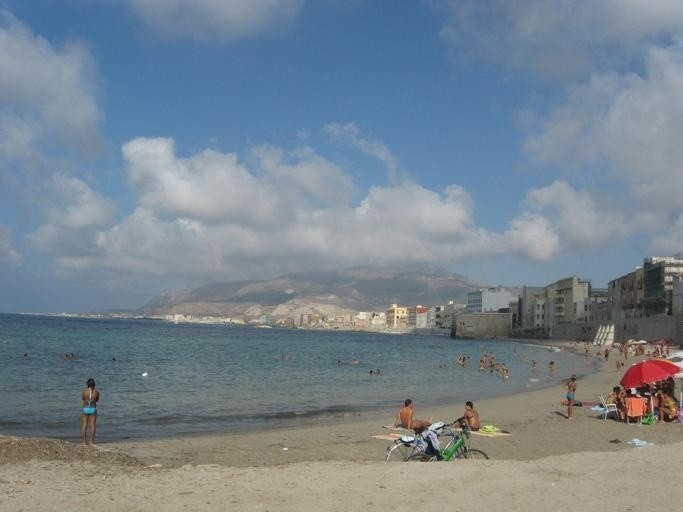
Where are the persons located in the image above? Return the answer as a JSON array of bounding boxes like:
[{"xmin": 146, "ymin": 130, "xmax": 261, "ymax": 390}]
[
  {"xmin": 456, "ymin": 351, "xmax": 509, "ymax": 384},
  {"xmin": 81, "ymin": 377, "xmax": 100, "ymax": 448},
  {"xmin": 451, "ymin": 400, "xmax": 480, "ymax": 431},
  {"xmin": 561, "ymin": 373, "xmax": 578, "ymax": 421},
  {"xmin": 391, "ymin": 398, "xmax": 433, "ymax": 431},
  {"xmin": 24, "ymin": 353, "xmax": 28, "ymax": 356},
  {"xmin": 338, "ymin": 359, "xmax": 381, "ymax": 375},
  {"xmin": 606, "ymin": 376, "xmax": 681, "ymax": 424},
  {"xmin": 531, "ymin": 359, "xmax": 537, "ymax": 369},
  {"xmin": 549, "ymin": 360, "xmax": 556, "ymax": 376},
  {"xmin": 111, "ymin": 357, "xmax": 116, "ymax": 361},
  {"xmin": 64, "ymin": 352, "xmax": 76, "ymax": 360},
  {"xmin": 616, "ymin": 359, "xmax": 624, "ymax": 372},
  {"xmin": 583, "ymin": 341, "xmax": 681, "ymax": 361}
]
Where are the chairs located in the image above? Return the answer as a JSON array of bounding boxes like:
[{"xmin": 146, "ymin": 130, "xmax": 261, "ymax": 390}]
[
  {"xmin": 625, "ymin": 398, "xmax": 645, "ymax": 425},
  {"xmin": 599, "ymin": 394, "xmax": 618, "ymax": 421}
]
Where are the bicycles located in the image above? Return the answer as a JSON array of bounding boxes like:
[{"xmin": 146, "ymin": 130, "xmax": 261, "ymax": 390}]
[{"xmin": 385, "ymin": 415, "xmax": 490, "ymax": 461}]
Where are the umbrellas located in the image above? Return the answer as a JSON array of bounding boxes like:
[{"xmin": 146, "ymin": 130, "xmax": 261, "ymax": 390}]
[
  {"xmin": 627, "ymin": 338, "xmax": 666, "ymax": 345},
  {"xmin": 619, "ymin": 358, "xmax": 682, "ymax": 417}
]
